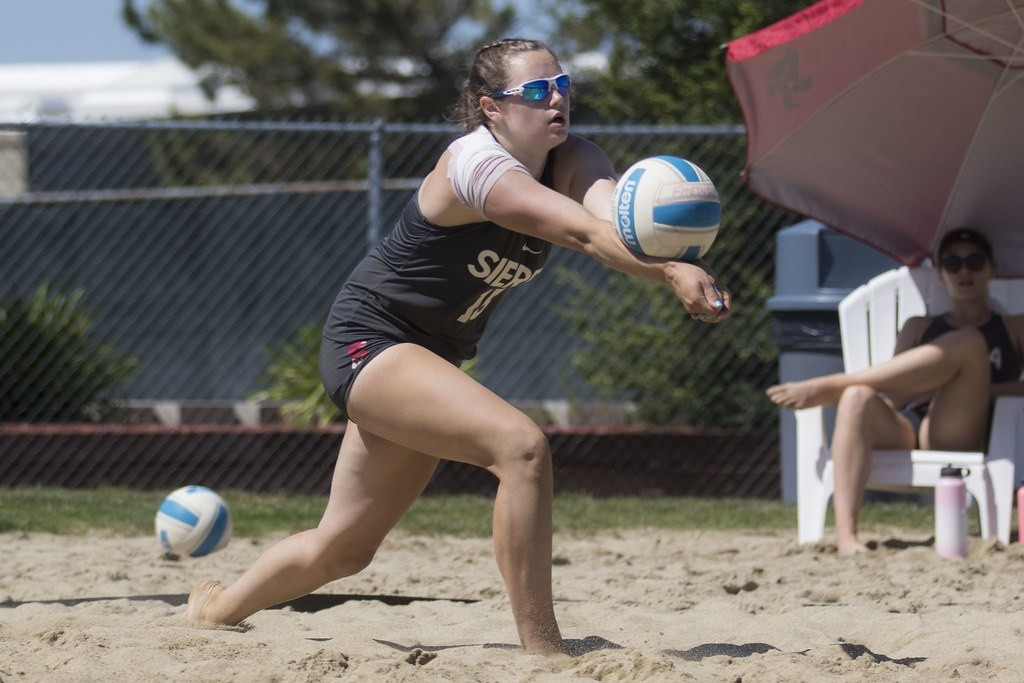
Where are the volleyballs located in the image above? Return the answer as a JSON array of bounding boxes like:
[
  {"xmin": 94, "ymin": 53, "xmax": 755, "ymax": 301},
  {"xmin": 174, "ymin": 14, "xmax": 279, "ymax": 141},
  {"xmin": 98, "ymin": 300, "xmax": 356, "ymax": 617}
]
[
  {"xmin": 612, "ymin": 156, "xmax": 721, "ymax": 261},
  {"xmin": 153, "ymin": 486, "xmax": 235, "ymax": 555}
]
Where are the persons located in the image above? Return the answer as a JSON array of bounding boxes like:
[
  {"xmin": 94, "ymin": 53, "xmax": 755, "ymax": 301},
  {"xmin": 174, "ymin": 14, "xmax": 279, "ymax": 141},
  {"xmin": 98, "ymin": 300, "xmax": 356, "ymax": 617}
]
[
  {"xmin": 763, "ymin": 226, "xmax": 1022, "ymax": 563},
  {"xmin": 183, "ymin": 38, "xmax": 735, "ymax": 659}
]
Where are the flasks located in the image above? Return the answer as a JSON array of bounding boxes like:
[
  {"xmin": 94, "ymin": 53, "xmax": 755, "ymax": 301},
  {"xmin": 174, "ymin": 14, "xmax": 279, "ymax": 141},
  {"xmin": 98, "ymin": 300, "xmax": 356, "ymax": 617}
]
[
  {"xmin": 1016, "ymin": 480, "xmax": 1023, "ymax": 547},
  {"xmin": 933, "ymin": 465, "xmax": 972, "ymax": 559}
]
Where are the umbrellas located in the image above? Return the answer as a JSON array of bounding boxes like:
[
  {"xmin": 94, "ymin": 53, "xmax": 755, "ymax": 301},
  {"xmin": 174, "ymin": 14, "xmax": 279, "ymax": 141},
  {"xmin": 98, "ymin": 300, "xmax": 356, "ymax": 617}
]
[{"xmin": 714, "ymin": 1, "xmax": 1023, "ymax": 279}]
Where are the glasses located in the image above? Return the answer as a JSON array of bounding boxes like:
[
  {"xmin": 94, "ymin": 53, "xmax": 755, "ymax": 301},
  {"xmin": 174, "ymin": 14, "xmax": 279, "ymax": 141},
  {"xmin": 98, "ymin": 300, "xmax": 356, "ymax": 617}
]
[
  {"xmin": 491, "ymin": 73, "xmax": 574, "ymax": 103},
  {"xmin": 940, "ymin": 252, "xmax": 992, "ymax": 274}
]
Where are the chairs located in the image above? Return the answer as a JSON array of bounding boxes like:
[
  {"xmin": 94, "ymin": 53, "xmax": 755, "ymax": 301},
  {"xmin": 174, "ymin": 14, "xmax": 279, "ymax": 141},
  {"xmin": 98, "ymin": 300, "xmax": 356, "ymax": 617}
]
[{"xmin": 795, "ymin": 257, "xmax": 1023, "ymax": 547}]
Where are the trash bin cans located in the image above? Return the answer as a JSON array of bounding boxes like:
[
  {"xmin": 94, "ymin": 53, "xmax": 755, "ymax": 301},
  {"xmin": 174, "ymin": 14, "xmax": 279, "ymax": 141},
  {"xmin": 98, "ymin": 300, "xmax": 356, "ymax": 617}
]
[{"xmin": 759, "ymin": 217, "xmax": 926, "ymax": 507}]
